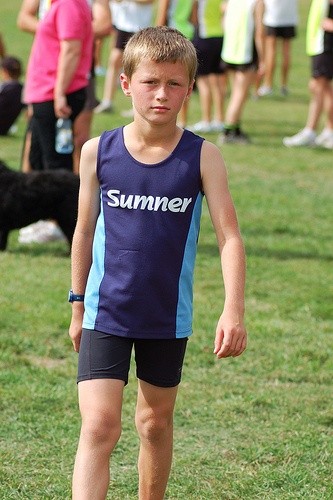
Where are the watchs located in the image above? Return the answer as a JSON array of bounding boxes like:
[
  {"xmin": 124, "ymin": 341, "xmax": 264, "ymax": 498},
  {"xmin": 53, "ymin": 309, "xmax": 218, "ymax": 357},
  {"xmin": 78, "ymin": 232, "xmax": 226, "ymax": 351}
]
[{"xmin": 66, "ymin": 290, "xmax": 83, "ymax": 303}]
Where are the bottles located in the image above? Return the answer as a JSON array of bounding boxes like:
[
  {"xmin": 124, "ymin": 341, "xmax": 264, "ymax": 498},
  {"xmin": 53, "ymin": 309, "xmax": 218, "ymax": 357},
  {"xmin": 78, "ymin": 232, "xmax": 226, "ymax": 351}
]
[{"xmin": 56, "ymin": 118, "xmax": 73, "ymax": 152}]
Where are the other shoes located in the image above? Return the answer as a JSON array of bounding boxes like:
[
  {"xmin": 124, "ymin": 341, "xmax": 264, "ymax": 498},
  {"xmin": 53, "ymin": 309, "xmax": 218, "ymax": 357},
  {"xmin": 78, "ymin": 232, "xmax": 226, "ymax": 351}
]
[
  {"xmin": 283, "ymin": 128, "xmax": 317, "ymax": 148},
  {"xmin": 91, "ymin": 100, "xmax": 112, "ymax": 114},
  {"xmin": 18, "ymin": 220, "xmax": 66, "ymax": 243},
  {"xmin": 307, "ymin": 129, "xmax": 333, "ymax": 149},
  {"xmin": 219, "ymin": 124, "xmax": 250, "ymax": 147},
  {"xmin": 187, "ymin": 121, "xmax": 225, "ymax": 133}
]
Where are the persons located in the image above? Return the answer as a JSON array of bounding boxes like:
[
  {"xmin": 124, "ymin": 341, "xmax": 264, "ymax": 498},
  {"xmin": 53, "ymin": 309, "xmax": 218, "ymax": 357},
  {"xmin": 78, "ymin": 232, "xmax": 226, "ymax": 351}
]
[
  {"xmin": 0, "ymin": 0, "xmax": 333, "ymax": 249},
  {"xmin": 68, "ymin": 28, "xmax": 247, "ymax": 500}
]
